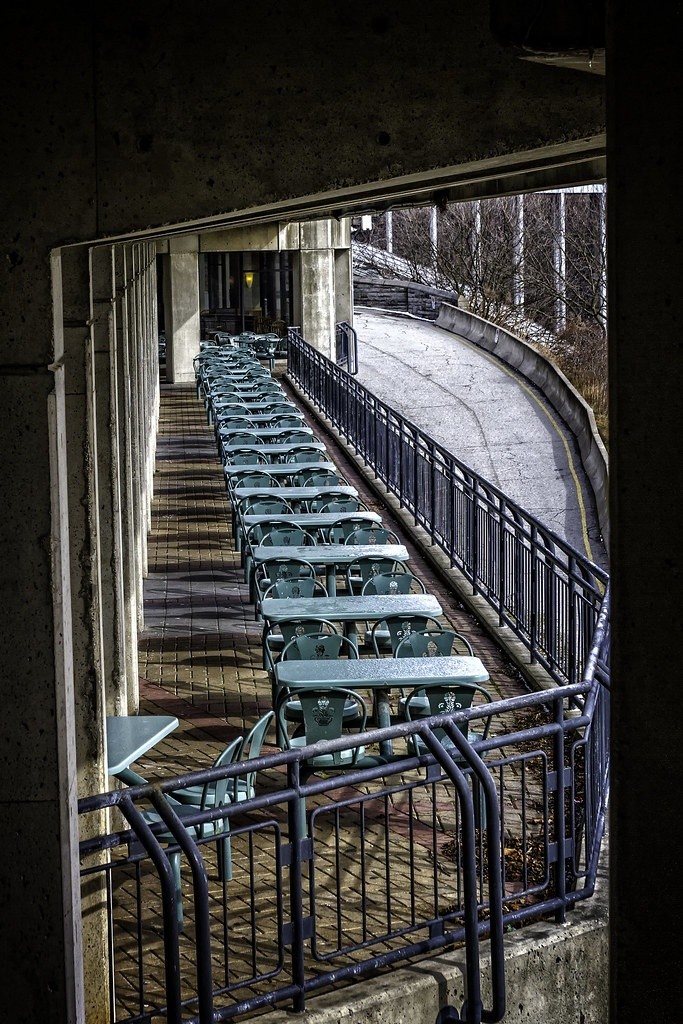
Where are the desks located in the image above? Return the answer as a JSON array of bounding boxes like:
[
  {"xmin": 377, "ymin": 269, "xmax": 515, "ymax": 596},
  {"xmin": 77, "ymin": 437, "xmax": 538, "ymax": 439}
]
[
  {"xmin": 224, "ymin": 462, "xmax": 337, "ymax": 500},
  {"xmin": 243, "ymin": 512, "xmax": 383, "ymax": 584},
  {"xmin": 204, "ymin": 374, "xmax": 271, "ymax": 409},
  {"xmin": 217, "ymin": 361, "xmax": 261, "ymax": 366},
  {"xmin": 213, "ymin": 401, "xmax": 296, "ymax": 436},
  {"xmin": 224, "ymin": 443, "xmax": 326, "ymax": 481},
  {"xmin": 233, "ymin": 486, "xmax": 358, "ymax": 551},
  {"xmin": 275, "ymin": 656, "xmax": 489, "ymax": 746},
  {"xmin": 234, "ymin": 337, "xmax": 283, "ymax": 371},
  {"xmin": 254, "ymin": 544, "xmax": 410, "ymax": 622},
  {"xmin": 208, "ymin": 391, "xmax": 287, "ymax": 426},
  {"xmin": 216, "ymin": 413, "xmax": 305, "ymax": 449},
  {"xmin": 197, "ymin": 369, "xmax": 270, "ymax": 400},
  {"xmin": 219, "ymin": 427, "xmax": 314, "ymax": 464},
  {"xmin": 107, "ymin": 715, "xmax": 182, "ymax": 806},
  {"xmin": 210, "ymin": 382, "xmax": 281, "ymax": 388},
  {"xmin": 259, "ymin": 594, "xmax": 443, "ymax": 670}
]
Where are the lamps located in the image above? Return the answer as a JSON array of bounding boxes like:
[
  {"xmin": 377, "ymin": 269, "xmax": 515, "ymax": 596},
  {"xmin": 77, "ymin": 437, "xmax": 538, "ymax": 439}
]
[{"xmin": 245, "ymin": 271, "xmax": 254, "ymax": 289}]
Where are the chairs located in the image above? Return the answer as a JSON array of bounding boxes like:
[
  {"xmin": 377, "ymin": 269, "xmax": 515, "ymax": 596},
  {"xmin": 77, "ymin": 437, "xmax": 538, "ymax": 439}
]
[
  {"xmin": 158, "ymin": 331, "xmax": 166, "ymax": 363},
  {"xmin": 194, "ymin": 312, "xmax": 495, "ymax": 829},
  {"xmin": 138, "ymin": 710, "xmax": 276, "ymax": 933}
]
